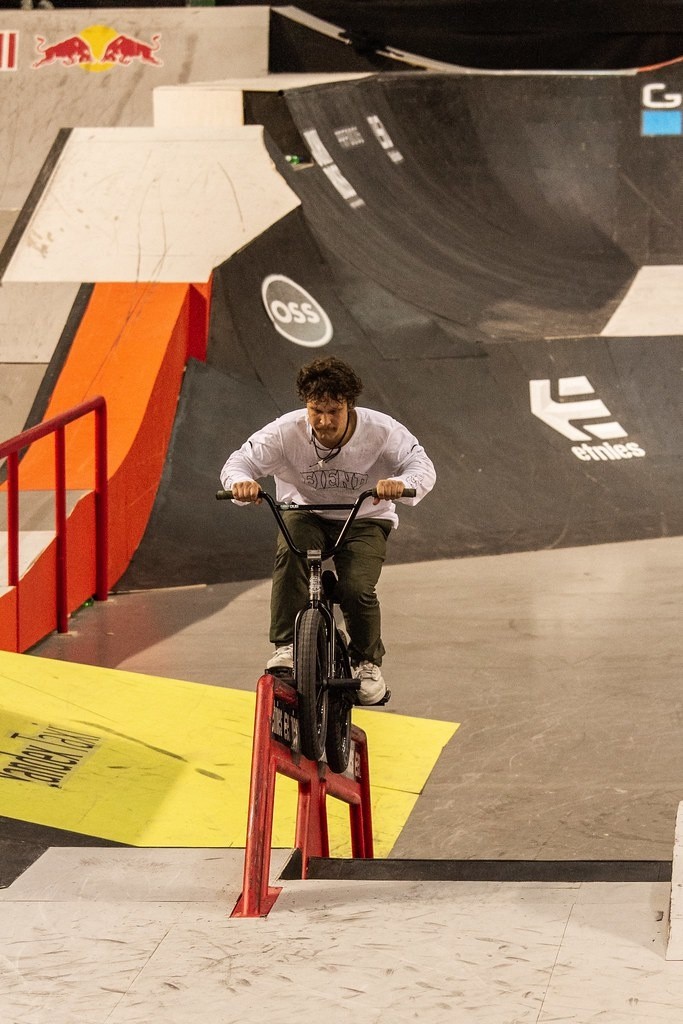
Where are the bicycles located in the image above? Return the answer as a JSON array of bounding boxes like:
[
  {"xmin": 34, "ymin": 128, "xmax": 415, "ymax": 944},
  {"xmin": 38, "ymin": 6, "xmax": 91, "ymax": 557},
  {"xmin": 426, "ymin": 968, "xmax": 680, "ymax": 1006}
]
[{"xmin": 211, "ymin": 485, "xmax": 418, "ymax": 775}]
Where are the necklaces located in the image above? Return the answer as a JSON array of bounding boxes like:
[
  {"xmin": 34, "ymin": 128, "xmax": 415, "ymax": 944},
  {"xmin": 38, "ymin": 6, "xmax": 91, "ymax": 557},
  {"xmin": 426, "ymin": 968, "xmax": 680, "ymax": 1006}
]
[{"xmin": 312, "ymin": 410, "xmax": 351, "ymax": 467}]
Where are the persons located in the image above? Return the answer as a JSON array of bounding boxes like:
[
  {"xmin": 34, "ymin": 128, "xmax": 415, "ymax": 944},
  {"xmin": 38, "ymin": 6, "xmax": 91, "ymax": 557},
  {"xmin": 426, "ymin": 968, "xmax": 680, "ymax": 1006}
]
[{"xmin": 219, "ymin": 354, "xmax": 439, "ymax": 707}]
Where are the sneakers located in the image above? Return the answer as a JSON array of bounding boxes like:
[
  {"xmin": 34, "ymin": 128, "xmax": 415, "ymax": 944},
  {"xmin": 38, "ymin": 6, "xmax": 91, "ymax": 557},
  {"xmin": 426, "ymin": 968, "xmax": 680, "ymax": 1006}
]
[{"xmin": 266, "ymin": 645, "xmax": 386, "ymax": 706}]
